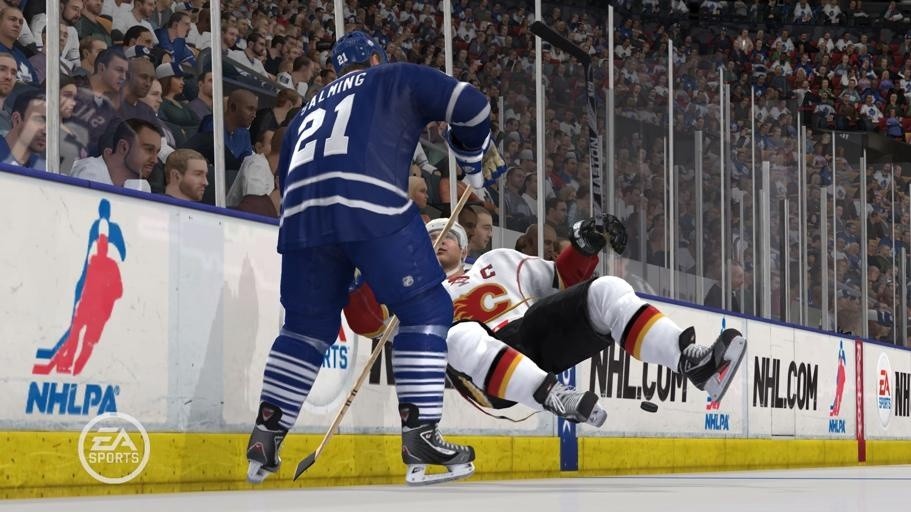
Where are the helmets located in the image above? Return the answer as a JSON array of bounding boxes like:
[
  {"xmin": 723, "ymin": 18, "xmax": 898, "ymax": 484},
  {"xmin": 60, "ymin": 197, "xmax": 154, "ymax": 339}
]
[
  {"xmin": 331, "ymin": 30, "xmax": 388, "ymax": 71},
  {"xmin": 426, "ymin": 218, "xmax": 468, "ymax": 252}
]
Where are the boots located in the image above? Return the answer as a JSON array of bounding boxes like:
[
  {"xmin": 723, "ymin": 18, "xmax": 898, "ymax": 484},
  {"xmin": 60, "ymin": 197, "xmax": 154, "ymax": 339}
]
[
  {"xmin": 533, "ymin": 371, "xmax": 598, "ymax": 422},
  {"xmin": 245, "ymin": 400, "xmax": 290, "ymax": 473},
  {"xmin": 397, "ymin": 402, "xmax": 475, "ymax": 464},
  {"xmin": 677, "ymin": 326, "xmax": 742, "ymax": 391}
]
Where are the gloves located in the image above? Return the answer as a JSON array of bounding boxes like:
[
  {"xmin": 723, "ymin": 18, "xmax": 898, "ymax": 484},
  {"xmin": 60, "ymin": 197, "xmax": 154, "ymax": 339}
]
[
  {"xmin": 567, "ymin": 214, "xmax": 627, "ymax": 258},
  {"xmin": 442, "ymin": 128, "xmax": 506, "ymax": 189}
]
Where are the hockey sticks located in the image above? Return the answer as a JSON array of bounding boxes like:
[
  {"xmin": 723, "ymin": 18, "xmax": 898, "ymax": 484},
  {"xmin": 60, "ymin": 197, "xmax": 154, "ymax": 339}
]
[
  {"xmin": 294, "ymin": 131, "xmax": 507, "ymax": 481},
  {"xmin": 530, "ymin": 20, "xmax": 604, "ymax": 232}
]
